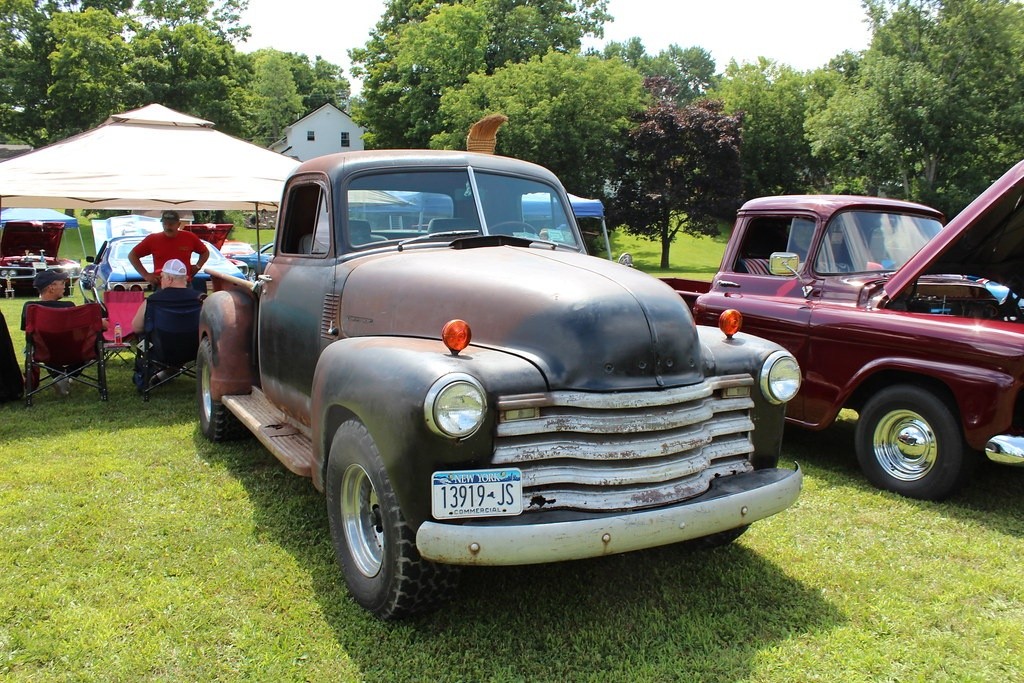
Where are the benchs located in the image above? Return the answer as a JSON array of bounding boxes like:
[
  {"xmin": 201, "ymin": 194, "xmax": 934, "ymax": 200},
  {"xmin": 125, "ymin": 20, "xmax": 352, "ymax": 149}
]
[{"xmin": 738, "ymin": 259, "xmax": 852, "ymax": 276}]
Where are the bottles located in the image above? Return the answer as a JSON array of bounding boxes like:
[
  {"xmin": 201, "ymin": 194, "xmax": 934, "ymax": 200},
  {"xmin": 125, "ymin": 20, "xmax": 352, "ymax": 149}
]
[{"xmin": 114, "ymin": 324, "xmax": 122, "ymax": 344}]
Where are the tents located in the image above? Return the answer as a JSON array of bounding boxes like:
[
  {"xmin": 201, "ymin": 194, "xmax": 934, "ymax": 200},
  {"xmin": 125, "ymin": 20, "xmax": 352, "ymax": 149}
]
[
  {"xmin": 0, "ymin": 208, "xmax": 87, "ymax": 258},
  {"xmin": 0, "ymin": 103, "xmax": 417, "ymax": 280},
  {"xmin": 346, "ymin": 188, "xmax": 613, "ymax": 261}
]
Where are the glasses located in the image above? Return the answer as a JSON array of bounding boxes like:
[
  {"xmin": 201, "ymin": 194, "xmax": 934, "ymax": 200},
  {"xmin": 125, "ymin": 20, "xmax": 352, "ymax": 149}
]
[{"xmin": 52, "ymin": 281, "xmax": 65, "ymax": 287}]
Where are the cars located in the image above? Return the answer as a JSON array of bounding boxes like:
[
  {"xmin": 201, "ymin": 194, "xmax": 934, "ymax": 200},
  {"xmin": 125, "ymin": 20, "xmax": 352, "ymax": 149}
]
[
  {"xmin": 80, "ymin": 213, "xmax": 257, "ymax": 319},
  {"xmin": 229, "ymin": 242, "xmax": 275, "ymax": 283},
  {"xmin": 196, "ymin": 146, "xmax": 804, "ymax": 621}
]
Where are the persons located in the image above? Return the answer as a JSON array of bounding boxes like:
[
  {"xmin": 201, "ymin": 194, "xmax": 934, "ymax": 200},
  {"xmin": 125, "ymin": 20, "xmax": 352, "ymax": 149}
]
[
  {"xmin": 20, "ymin": 271, "xmax": 109, "ymax": 396},
  {"xmin": 127, "ymin": 210, "xmax": 210, "ymax": 293},
  {"xmin": 131, "ymin": 259, "xmax": 205, "ymax": 385}
]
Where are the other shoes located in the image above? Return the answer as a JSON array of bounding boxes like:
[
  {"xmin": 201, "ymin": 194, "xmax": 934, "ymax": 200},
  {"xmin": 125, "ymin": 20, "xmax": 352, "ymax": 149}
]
[
  {"xmin": 52, "ymin": 376, "xmax": 69, "ymax": 396},
  {"xmin": 149, "ymin": 374, "xmax": 165, "ymax": 389}
]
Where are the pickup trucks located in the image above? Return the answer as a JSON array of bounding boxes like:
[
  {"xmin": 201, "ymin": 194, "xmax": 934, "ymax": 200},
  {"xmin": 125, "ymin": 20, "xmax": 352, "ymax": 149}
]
[{"xmin": 657, "ymin": 158, "xmax": 1024, "ymax": 501}]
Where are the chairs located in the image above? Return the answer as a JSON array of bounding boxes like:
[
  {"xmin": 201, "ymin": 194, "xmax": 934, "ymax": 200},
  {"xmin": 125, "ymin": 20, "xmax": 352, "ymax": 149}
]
[
  {"xmin": 142, "ymin": 298, "xmax": 205, "ymax": 403},
  {"xmin": 24, "ymin": 303, "xmax": 108, "ymax": 408},
  {"xmin": 427, "ymin": 217, "xmax": 469, "ymax": 235},
  {"xmin": 101, "ymin": 291, "xmax": 145, "ymax": 367},
  {"xmin": 348, "ymin": 220, "xmax": 372, "ymax": 245}
]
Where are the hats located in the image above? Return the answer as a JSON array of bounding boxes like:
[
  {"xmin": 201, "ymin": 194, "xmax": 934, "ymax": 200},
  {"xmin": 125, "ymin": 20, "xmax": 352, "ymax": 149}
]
[
  {"xmin": 33, "ymin": 269, "xmax": 68, "ymax": 289},
  {"xmin": 155, "ymin": 259, "xmax": 187, "ymax": 275},
  {"xmin": 160, "ymin": 211, "xmax": 180, "ymax": 222}
]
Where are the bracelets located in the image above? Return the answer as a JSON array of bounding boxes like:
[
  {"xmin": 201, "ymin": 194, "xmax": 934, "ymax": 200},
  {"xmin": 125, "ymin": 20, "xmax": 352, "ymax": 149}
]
[{"xmin": 196, "ymin": 265, "xmax": 200, "ymax": 270}]
